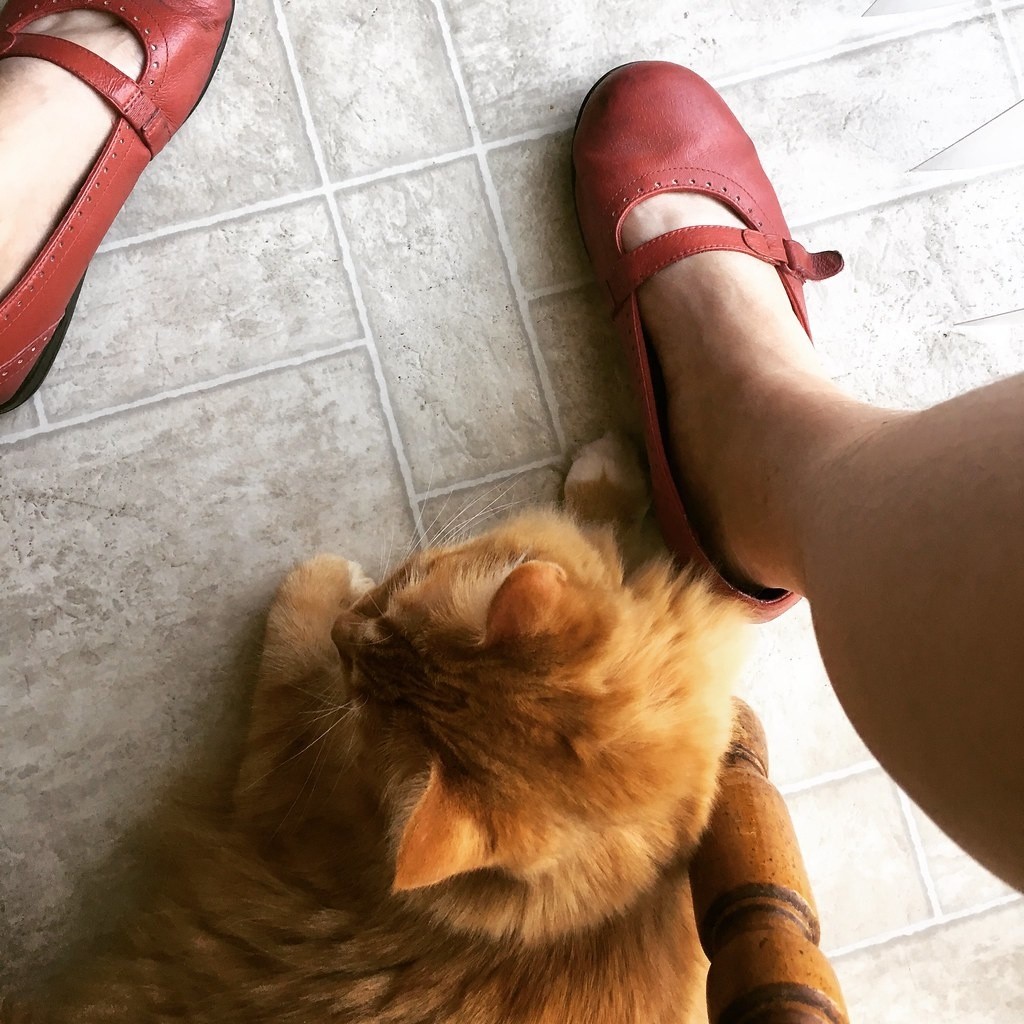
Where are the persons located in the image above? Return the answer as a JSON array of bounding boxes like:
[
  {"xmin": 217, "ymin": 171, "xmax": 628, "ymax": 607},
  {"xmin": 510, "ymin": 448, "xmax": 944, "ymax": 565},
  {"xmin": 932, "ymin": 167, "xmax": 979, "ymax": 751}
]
[{"xmin": 0, "ymin": 0, "xmax": 1024, "ymax": 897}]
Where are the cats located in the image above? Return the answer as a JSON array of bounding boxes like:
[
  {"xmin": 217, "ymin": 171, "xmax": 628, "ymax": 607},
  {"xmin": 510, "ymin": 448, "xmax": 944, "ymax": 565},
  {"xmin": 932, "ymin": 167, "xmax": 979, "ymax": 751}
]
[{"xmin": 0, "ymin": 424, "xmax": 752, "ymax": 1023}]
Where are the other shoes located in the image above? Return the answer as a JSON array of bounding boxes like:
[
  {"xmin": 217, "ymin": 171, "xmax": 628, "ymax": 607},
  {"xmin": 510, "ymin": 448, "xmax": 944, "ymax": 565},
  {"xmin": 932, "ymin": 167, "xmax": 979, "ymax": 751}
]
[
  {"xmin": 0, "ymin": 1, "xmax": 234, "ymax": 413},
  {"xmin": 573, "ymin": 62, "xmax": 846, "ymax": 621}
]
